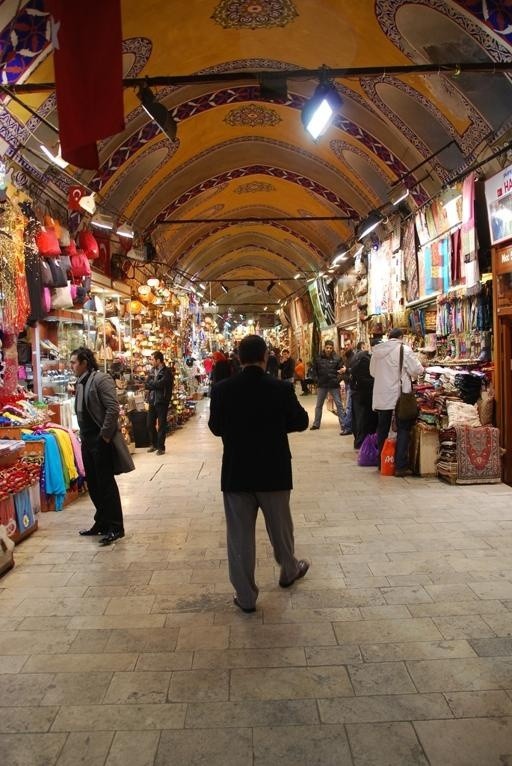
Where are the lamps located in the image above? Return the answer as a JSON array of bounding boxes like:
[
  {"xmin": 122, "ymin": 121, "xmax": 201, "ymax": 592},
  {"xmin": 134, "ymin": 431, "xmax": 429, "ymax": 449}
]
[
  {"xmin": 90, "ymin": 214, "xmax": 113, "ymax": 229},
  {"xmin": 300, "ymin": 75, "xmax": 344, "ymax": 141},
  {"xmin": 358, "ymin": 209, "xmax": 383, "ymax": 240},
  {"xmin": 331, "ymin": 243, "xmax": 349, "ymax": 266},
  {"xmin": 117, "ymin": 222, "xmax": 134, "ymax": 239},
  {"xmin": 144, "ymin": 241, "xmax": 156, "ymax": 265},
  {"xmin": 136, "ymin": 86, "xmax": 177, "ymax": 142},
  {"xmin": 390, "ymin": 189, "xmax": 410, "ymax": 204}
]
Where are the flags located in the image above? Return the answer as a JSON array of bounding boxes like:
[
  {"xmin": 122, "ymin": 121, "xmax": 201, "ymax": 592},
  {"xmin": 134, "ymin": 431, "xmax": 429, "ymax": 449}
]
[{"xmin": 49, "ymin": 0, "xmax": 126, "ymax": 169}]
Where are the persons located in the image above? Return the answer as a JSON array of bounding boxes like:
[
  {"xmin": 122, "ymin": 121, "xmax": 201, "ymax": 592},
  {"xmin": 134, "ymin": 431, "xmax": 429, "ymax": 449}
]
[
  {"xmin": 336, "ymin": 337, "xmax": 385, "ymax": 437},
  {"xmin": 310, "ymin": 341, "xmax": 346, "ymax": 432},
  {"xmin": 208, "ymin": 334, "xmax": 310, "ymax": 614},
  {"xmin": 280, "ymin": 350, "xmax": 295, "ymax": 382},
  {"xmin": 300, "ymin": 363, "xmax": 317, "ymax": 395},
  {"xmin": 70, "ymin": 346, "xmax": 135, "ymax": 544},
  {"xmin": 267, "ymin": 351, "xmax": 277, "ymax": 377},
  {"xmin": 295, "ymin": 359, "xmax": 305, "ymax": 380},
  {"xmin": 370, "ymin": 329, "xmax": 424, "ymax": 476},
  {"xmin": 208, "ymin": 340, "xmax": 242, "ymax": 397},
  {"xmin": 143, "ymin": 351, "xmax": 174, "ymax": 455}
]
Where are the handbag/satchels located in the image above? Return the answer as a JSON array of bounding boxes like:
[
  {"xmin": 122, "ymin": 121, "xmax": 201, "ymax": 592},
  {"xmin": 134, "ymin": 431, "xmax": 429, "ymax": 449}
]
[
  {"xmin": 380, "ymin": 437, "xmax": 395, "ymax": 477},
  {"xmin": 41, "ymin": 288, "xmax": 49, "ymax": 313},
  {"xmin": 57, "ymin": 228, "xmax": 70, "ymax": 246},
  {"xmin": 44, "ymin": 201, "xmax": 62, "ymax": 237},
  {"xmin": 61, "ymin": 257, "xmax": 73, "ymax": 279},
  {"xmin": 71, "ymin": 249, "xmax": 91, "ymax": 278},
  {"xmin": 395, "ymin": 343, "xmax": 418, "ymax": 421},
  {"xmin": 50, "ymin": 259, "xmax": 67, "ymax": 288},
  {"xmin": 64, "ymin": 240, "xmax": 78, "ymax": 256},
  {"xmin": 40, "ymin": 261, "xmax": 52, "ymax": 286},
  {"xmin": 38, "ymin": 228, "xmax": 60, "ymax": 256},
  {"xmin": 80, "ymin": 231, "xmax": 99, "ymax": 258},
  {"xmin": 50, "ymin": 281, "xmax": 73, "ymax": 310},
  {"xmin": 350, "ymin": 354, "xmax": 370, "ymax": 390},
  {"xmin": 358, "ymin": 434, "xmax": 379, "ymax": 466}
]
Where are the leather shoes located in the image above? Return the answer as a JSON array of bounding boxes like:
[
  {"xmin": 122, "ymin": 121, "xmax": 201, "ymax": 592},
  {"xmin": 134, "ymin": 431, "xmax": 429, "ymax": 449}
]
[
  {"xmin": 98, "ymin": 528, "xmax": 124, "ymax": 543},
  {"xmin": 279, "ymin": 559, "xmax": 309, "ymax": 586},
  {"xmin": 147, "ymin": 447, "xmax": 157, "ymax": 452},
  {"xmin": 310, "ymin": 425, "xmax": 318, "ymax": 430},
  {"xmin": 340, "ymin": 430, "xmax": 351, "ymax": 435},
  {"xmin": 395, "ymin": 468, "xmax": 413, "ymax": 477},
  {"xmin": 233, "ymin": 591, "xmax": 256, "ymax": 612},
  {"xmin": 156, "ymin": 449, "xmax": 164, "ymax": 455},
  {"xmin": 79, "ymin": 523, "xmax": 108, "ymax": 535}
]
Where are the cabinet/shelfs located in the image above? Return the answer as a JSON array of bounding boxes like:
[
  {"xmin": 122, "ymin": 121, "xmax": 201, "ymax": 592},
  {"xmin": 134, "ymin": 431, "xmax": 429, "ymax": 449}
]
[
  {"xmin": 95, "ymin": 277, "xmax": 246, "ymax": 456},
  {"xmin": 265, "ymin": 252, "xmax": 355, "ymax": 427},
  {"xmin": 0, "ymin": 174, "xmax": 80, "ymax": 575},
  {"xmin": 356, "ymin": 166, "xmax": 490, "ymax": 469}
]
